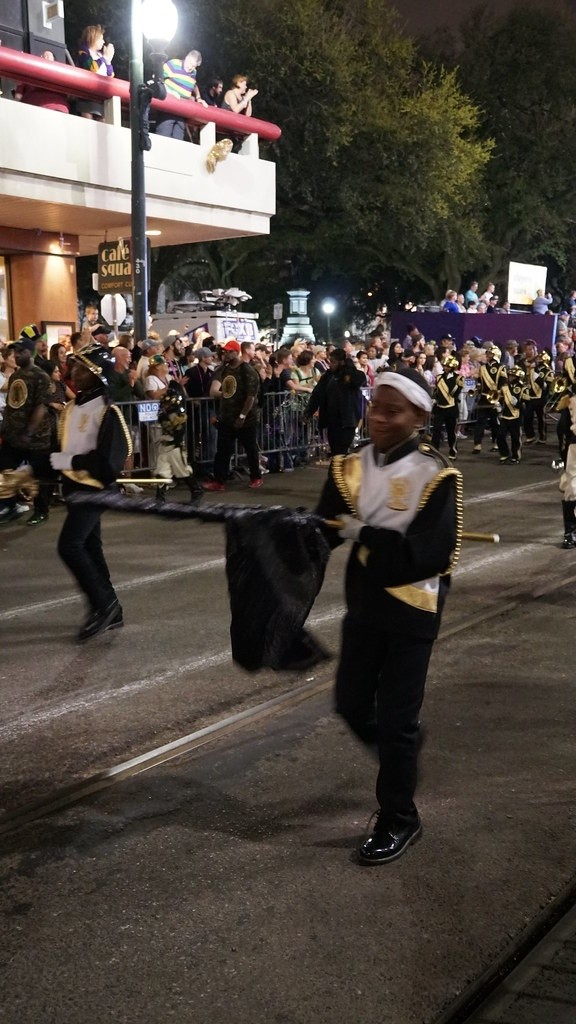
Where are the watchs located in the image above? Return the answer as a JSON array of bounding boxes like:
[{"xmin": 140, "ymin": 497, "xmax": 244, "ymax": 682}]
[{"xmin": 240, "ymin": 414, "xmax": 246, "ymax": 419}]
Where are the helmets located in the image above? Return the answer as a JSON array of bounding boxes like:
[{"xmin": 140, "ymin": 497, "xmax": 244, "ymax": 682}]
[{"xmin": 442, "ymin": 344, "xmax": 552, "ymax": 385}]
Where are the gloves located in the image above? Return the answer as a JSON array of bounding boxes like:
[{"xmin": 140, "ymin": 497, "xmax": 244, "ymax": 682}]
[
  {"xmin": 336, "ymin": 514, "xmax": 367, "ymax": 543},
  {"xmin": 50, "ymin": 452, "xmax": 74, "ymax": 471}
]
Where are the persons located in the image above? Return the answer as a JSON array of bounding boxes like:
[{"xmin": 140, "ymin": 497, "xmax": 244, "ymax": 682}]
[
  {"xmin": 15, "ymin": 24, "xmax": 115, "ymax": 122},
  {"xmin": 304, "ymin": 349, "xmax": 366, "ymax": 456},
  {"xmin": 0, "ymin": 305, "xmax": 340, "ymax": 526},
  {"xmin": 51, "ymin": 352, "xmax": 133, "ymax": 643},
  {"xmin": 147, "ymin": 50, "xmax": 259, "ymax": 154},
  {"xmin": 343, "ymin": 281, "xmax": 576, "ymax": 549},
  {"xmin": 310, "ymin": 368, "xmax": 463, "ymax": 865}
]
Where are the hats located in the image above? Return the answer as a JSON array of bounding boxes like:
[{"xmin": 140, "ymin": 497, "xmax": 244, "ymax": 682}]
[
  {"xmin": 7, "ymin": 338, "xmax": 36, "ymax": 355},
  {"xmin": 442, "ymin": 333, "xmax": 456, "ymax": 341},
  {"xmin": 91, "ymin": 326, "xmax": 111, "ymax": 337},
  {"xmin": 222, "ymin": 341, "xmax": 240, "ymax": 355},
  {"xmin": 141, "ymin": 339, "xmax": 156, "ymax": 353},
  {"xmin": 160, "ymin": 380, "xmax": 187, "ymax": 415},
  {"xmin": 18, "ymin": 324, "xmax": 42, "ymax": 342},
  {"xmin": 148, "ymin": 354, "xmax": 169, "ymax": 368},
  {"xmin": 64, "ymin": 344, "xmax": 115, "ymax": 388},
  {"xmin": 198, "ymin": 347, "xmax": 215, "ymax": 357},
  {"xmin": 162, "ymin": 335, "xmax": 178, "ymax": 353},
  {"xmin": 490, "ymin": 297, "xmax": 498, "ymax": 300}
]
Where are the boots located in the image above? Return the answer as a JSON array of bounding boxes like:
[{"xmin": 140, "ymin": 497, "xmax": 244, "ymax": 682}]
[
  {"xmin": 182, "ymin": 475, "xmax": 205, "ymax": 506},
  {"xmin": 154, "ymin": 475, "xmax": 166, "ymax": 502},
  {"xmin": 562, "ymin": 499, "xmax": 576, "ymax": 548}
]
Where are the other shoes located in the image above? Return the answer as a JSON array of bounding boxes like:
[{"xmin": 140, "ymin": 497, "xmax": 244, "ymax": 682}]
[
  {"xmin": 0, "ymin": 503, "xmax": 29, "ymax": 515},
  {"xmin": 444, "ymin": 436, "xmax": 564, "ymax": 469}
]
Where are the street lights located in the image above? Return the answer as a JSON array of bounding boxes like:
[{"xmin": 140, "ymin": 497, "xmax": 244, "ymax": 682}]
[
  {"xmin": 323, "ymin": 296, "xmax": 339, "ymax": 345},
  {"xmin": 129, "ymin": 0, "xmax": 182, "ymax": 477}
]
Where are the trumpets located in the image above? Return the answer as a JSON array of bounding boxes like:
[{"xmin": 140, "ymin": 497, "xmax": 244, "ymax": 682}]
[{"xmin": 468, "ymin": 387, "xmax": 482, "ymax": 398}]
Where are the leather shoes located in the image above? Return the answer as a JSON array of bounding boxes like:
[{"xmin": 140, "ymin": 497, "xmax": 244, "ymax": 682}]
[
  {"xmin": 0, "ymin": 510, "xmax": 16, "ymax": 523},
  {"xmin": 358, "ymin": 809, "xmax": 423, "ymax": 866},
  {"xmin": 78, "ymin": 596, "xmax": 124, "ymax": 644},
  {"xmin": 27, "ymin": 512, "xmax": 49, "ymax": 526}
]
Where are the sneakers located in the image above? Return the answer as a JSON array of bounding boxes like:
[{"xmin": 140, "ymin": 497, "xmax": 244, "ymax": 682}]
[
  {"xmin": 203, "ymin": 481, "xmax": 225, "ymax": 491},
  {"xmin": 248, "ymin": 479, "xmax": 263, "ymax": 488}
]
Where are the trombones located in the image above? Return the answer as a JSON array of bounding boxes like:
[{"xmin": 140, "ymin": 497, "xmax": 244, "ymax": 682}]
[
  {"xmin": 487, "ymin": 380, "xmax": 511, "ymax": 404},
  {"xmin": 544, "ymin": 375, "xmax": 568, "ymax": 412}
]
